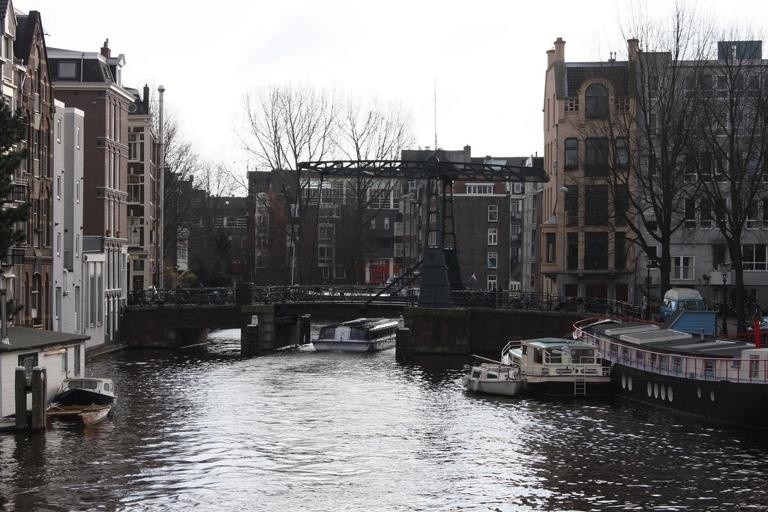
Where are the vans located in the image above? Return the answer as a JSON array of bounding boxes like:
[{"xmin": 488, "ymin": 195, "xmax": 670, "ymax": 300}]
[{"xmin": 659, "ymin": 287, "xmax": 704, "ymax": 322}]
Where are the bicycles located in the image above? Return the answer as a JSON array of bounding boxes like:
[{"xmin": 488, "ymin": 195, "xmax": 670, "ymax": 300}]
[
  {"xmin": 132, "ymin": 287, "xmax": 325, "ymax": 304},
  {"xmin": 452, "ymin": 290, "xmax": 639, "ymax": 319}
]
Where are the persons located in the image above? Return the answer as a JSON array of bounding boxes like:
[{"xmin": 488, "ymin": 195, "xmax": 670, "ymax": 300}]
[{"xmin": 722, "ymin": 318, "xmax": 728, "ymax": 335}]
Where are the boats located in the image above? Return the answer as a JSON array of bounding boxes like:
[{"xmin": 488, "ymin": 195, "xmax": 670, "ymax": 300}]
[
  {"xmin": 463, "ymin": 363, "xmax": 523, "ymax": 397},
  {"xmin": 500, "ymin": 336, "xmax": 612, "ymax": 398},
  {"xmin": 312, "ymin": 317, "xmax": 399, "ymax": 353},
  {"xmin": 45, "ymin": 376, "xmax": 118, "ymax": 428}
]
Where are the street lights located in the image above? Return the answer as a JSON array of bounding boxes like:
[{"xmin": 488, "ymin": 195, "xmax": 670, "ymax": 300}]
[{"xmin": 720, "ymin": 271, "xmax": 728, "ymax": 340}]
